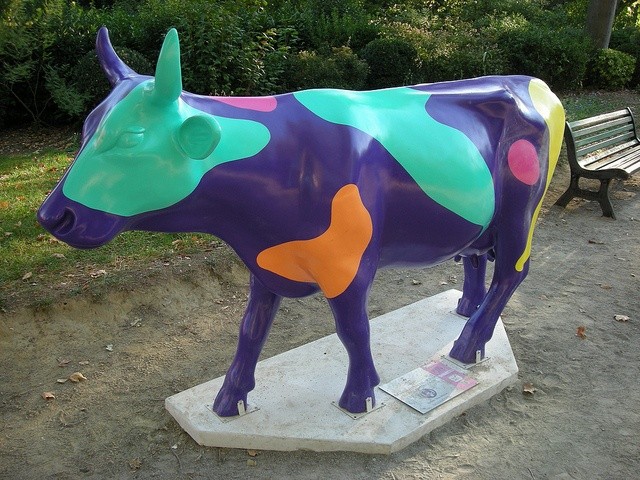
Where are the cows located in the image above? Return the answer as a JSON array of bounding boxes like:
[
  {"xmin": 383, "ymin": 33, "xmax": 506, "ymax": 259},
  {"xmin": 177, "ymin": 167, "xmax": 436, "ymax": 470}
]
[{"xmin": 37, "ymin": 26, "xmax": 566, "ymax": 417}]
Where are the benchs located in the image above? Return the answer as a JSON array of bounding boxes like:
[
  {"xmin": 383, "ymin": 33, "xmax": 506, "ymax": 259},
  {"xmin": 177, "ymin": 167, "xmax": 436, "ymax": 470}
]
[{"xmin": 553, "ymin": 106, "xmax": 640, "ymax": 220}]
